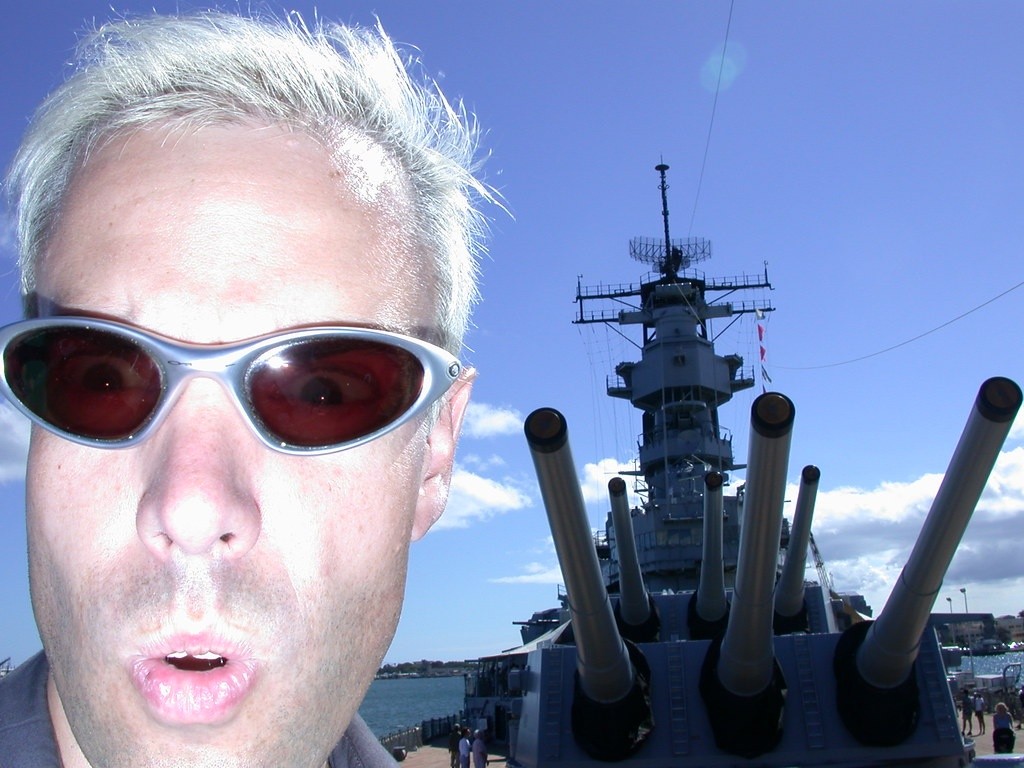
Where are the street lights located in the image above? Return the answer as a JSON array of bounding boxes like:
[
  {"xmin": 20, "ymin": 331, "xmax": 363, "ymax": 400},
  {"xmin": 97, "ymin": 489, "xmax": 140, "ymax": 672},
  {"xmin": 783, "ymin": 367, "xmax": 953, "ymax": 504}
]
[
  {"xmin": 945, "ymin": 598, "xmax": 955, "ymax": 645},
  {"xmin": 959, "ymin": 588, "xmax": 975, "ymax": 679}
]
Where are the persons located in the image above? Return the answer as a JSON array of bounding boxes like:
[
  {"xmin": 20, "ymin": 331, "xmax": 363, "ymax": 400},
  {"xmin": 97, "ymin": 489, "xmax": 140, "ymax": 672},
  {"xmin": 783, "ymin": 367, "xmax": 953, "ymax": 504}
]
[
  {"xmin": 0, "ymin": 9, "xmax": 516, "ymax": 768},
  {"xmin": 953, "ymin": 683, "xmax": 1024, "ymax": 754}
]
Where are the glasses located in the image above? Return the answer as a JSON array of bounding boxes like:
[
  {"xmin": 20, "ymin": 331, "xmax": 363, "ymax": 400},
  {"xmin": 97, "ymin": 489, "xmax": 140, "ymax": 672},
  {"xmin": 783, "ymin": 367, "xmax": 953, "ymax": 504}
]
[{"xmin": 0, "ymin": 315, "xmax": 462, "ymax": 455}]
